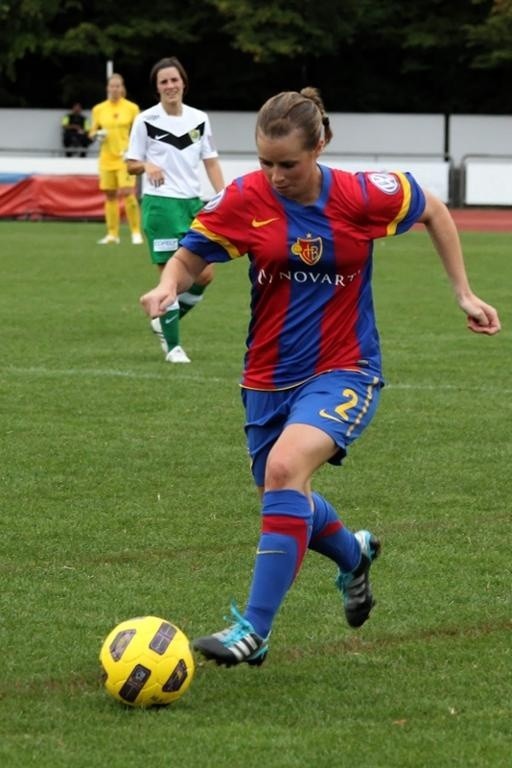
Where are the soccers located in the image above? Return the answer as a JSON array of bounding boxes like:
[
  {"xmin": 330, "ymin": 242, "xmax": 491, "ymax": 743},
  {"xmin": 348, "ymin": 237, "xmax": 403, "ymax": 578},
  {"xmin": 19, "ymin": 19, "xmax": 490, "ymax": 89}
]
[{"xmin": 99, "ymin": 615, "xmax": 194, "ymax": 706}]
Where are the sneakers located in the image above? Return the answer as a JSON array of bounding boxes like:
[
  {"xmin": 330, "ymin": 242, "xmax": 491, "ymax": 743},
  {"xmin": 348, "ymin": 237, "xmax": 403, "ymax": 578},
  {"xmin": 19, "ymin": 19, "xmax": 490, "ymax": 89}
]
[
  {"xmin": 97, "ymin": 236, "xmax": 120, "ymax": 246},
  {"xmin": 150, "ymin": 318, "xmax": 168, "ymax": 352},
  {"xmin": 191, "ymin": 607, "xmax": 267, "ymax": 668},
  {"xmin": 132, "ymin": 234, "xmax": 142, "ymax": 245},
  {"xmin": 337, "ymin": 530, "xmax": 381, "ymax": 627},
  {"xmin": 165, "ymin": 347, "xmax": 189, "ymax": 363}
]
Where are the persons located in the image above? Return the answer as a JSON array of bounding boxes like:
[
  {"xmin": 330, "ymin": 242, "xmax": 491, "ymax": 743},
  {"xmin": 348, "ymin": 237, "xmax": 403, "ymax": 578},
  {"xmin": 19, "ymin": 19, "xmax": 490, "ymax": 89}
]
[
  {"xmin": 62, "ymin": 101, "xmax": 94, "ymax": 157},
  {"xmin": 127, "ymin": 56, "xmax": 225, "ymax": 360},
  {"xmin": 90, "ymin": 72, "xmax": 144, "ymax": 246},
  {"xmin": 141, "ymin": 87, "xmax": 502, "ymax": 666}
]
